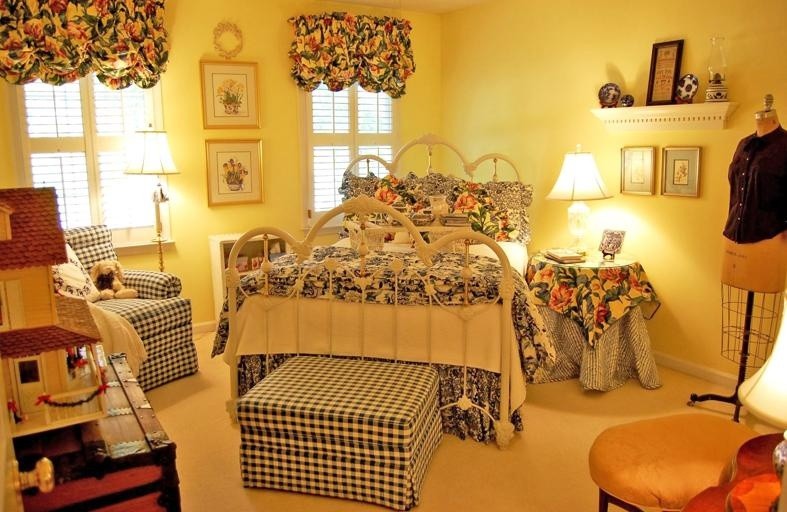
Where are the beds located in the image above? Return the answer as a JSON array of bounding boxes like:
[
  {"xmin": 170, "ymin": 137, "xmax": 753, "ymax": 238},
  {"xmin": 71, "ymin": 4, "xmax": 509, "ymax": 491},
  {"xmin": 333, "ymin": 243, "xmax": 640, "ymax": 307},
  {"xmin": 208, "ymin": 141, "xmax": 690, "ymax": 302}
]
[{"xmin": 211, "ymin": 134, "xmax": 557, "ymax": 449}]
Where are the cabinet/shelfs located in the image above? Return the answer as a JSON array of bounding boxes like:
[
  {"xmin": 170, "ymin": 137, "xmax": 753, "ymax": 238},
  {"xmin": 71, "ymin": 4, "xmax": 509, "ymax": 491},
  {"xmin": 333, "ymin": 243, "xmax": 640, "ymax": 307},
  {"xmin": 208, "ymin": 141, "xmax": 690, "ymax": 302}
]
[{"xmin": 17, "ymin": 354, "xmax": 180, "ymax": 511}]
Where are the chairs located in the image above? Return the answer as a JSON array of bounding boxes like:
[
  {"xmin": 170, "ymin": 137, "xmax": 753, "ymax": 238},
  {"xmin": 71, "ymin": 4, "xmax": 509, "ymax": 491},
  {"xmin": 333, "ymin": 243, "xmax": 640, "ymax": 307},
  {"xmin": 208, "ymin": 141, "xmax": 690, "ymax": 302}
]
[{"xmin": 52, "ymin": 223, "xmax": 198, "ymax": 393}]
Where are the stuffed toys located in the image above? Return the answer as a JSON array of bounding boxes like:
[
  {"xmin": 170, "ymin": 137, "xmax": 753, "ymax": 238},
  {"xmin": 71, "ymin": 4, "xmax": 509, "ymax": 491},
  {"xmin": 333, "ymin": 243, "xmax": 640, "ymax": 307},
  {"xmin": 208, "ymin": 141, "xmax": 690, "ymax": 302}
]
[{"xmin": 88, "ymin": 260, "xmax": 137, "ymax": 300}]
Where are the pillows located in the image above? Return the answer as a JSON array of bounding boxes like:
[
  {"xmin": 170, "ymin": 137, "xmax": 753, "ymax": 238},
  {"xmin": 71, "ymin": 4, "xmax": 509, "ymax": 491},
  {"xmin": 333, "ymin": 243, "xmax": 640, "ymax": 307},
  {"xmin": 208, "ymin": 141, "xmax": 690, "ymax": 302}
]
[
  {"xmin": 340, "ymin": 174, "xmax": 533, "ymax": 248},
  {"xmin": 52, "ymin": 243, "xmax": 102, "ymax": 305}
]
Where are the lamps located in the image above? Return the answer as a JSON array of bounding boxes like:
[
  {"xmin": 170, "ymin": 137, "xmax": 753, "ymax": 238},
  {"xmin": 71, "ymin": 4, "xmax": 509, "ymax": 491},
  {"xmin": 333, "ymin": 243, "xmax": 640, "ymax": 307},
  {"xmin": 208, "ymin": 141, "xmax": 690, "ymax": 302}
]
[
  {"xmin": 546, "ymin": 146, "xmax": 613, "ymax": 255},
  {"xmin": 737, "ymin": 291, "xmax": 787, "ymax": 436},
  {"xmin": 704, "ymin": 38, "xmax": 726, "ymax": 102},
  {"xmin": 125, "ymin": 124, "xmax": 182, "ymax": 243}
]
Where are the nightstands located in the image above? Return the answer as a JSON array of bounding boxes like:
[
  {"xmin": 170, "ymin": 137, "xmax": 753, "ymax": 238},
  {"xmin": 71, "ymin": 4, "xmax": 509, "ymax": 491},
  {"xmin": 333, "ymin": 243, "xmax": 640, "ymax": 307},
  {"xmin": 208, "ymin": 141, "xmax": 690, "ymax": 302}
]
[{"xmin": 210, "ymin": 233, "xmax": 287, "ymax": 322}]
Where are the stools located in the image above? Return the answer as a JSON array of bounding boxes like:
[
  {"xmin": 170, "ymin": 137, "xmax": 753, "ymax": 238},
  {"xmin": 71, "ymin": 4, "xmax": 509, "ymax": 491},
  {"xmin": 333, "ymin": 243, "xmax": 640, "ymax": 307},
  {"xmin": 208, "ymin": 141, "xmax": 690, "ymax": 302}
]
[
  {"xmin": 589, "ymin": 413, "xmax": 764, "ymax": 512},
  {"xmin": 238, "ymin": 356, "xmax": 442, "ymax": 511}
]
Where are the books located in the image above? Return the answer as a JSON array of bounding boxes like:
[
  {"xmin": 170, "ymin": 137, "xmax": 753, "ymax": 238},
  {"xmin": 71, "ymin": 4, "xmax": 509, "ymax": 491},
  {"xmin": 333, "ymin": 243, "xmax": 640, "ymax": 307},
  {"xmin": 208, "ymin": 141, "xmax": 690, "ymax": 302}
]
[
  {"xmin": 438, "ymin": 212, "xmax": 472, "ymax": 226},
  {"xmin": 543, "ymin": 247, "xmax": 587, "ymax": 265}
]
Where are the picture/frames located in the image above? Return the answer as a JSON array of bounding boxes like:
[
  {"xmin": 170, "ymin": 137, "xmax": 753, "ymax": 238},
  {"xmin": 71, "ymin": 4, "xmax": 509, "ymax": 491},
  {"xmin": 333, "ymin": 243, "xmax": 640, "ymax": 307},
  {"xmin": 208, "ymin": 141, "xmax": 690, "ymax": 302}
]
[
  {"xmin": 646, "ymin": 39, "xmax": 684, "ymax": 104},
  {"xmin": 599, "ymin": 229, "xmax": 625, "ymax": 262},
  {"xmin": 661, "ymin": 145, "xmax": 701, "ymax": 198},
  {"xmin": 199, "ymin": 59, "xmax": 262, "ymax": 130},
  {"xmin": 619, "ymin": 146, "xmax": 656, "ymax": 195},
  {"xmin": 205, "ymin": 138, "xmax": 265, "ymax": 207}
]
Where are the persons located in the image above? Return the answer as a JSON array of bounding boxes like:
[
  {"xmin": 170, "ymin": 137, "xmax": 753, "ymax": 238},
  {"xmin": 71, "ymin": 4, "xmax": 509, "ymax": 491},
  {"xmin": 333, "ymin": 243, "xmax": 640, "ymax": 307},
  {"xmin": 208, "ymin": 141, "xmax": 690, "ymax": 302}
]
[{"xmin": 721, "ymin": 110, "xmax": 787, "ymax": 293}]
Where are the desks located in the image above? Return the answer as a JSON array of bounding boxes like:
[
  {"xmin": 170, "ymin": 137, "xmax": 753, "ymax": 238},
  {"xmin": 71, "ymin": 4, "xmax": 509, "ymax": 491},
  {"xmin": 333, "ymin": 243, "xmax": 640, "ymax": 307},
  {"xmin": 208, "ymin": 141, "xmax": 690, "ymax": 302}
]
[
  {"xmin": 526, "ymin": 250, "xmax": 663, "ymax": 394},
  {"xmin": 664, "ymin": 430, "xmax": 786, "ymax": 511}
]
